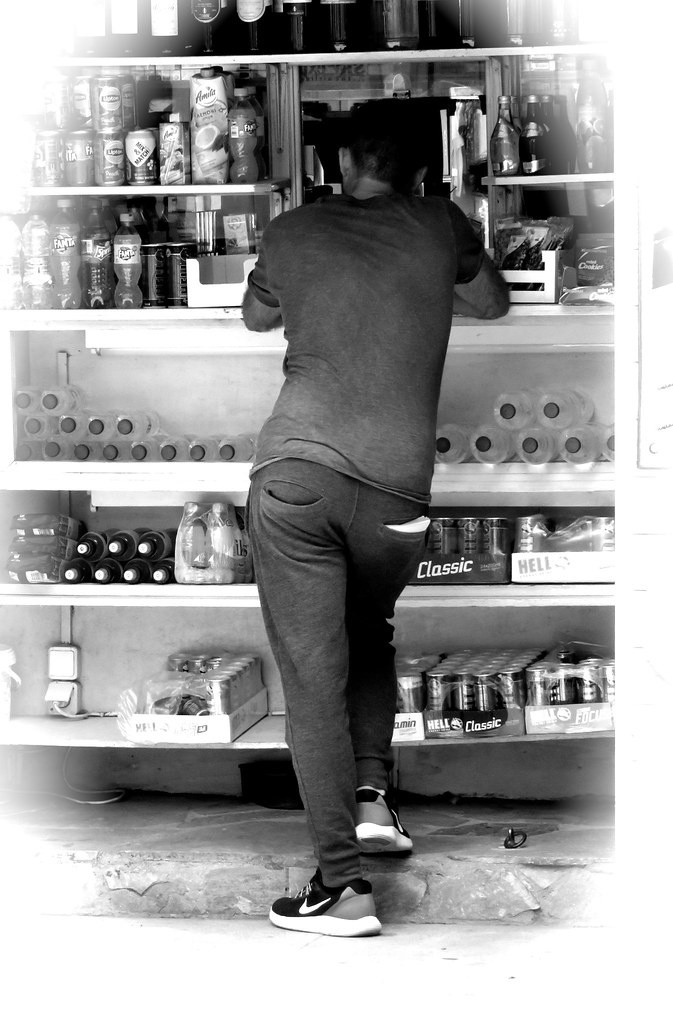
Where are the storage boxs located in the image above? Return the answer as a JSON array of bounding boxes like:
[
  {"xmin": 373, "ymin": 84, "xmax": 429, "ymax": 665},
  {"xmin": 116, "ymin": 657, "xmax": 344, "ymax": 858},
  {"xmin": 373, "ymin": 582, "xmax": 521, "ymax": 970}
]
[
  {"xmin": 511, "ymin": 551, "xmax": 616, "ymax": 583},
  {"xmin": 423, "ymin": 704, "xmax": 524, "ymax": 739},
  {"xmin": 408, "ymin": 552, "xmax": 510, "ymax": 583},
  {"xmin": 129, "ymin": 687, "xmax": 268, "ymax": 744},
  {"xmin": 525, "ymin": 700, "xmax": 615, "ymax": 735},
  {"xmin": 486, "ymin": 247, "xmax": 576, "ymax": 304},
  {"xmin": 186, "ymin": 254, "xmax": 259, "ymax": 308},
  {"xmin": 222, "ymin": 213, "xmax": 251, "ymax": 254}
]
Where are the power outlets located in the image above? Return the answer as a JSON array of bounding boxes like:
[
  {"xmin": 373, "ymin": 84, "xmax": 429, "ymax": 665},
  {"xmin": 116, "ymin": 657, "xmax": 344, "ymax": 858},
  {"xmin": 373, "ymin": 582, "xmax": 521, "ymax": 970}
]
[{"xmin": 45, "ymin": 681, "xmax": 82, "ymax": 716}]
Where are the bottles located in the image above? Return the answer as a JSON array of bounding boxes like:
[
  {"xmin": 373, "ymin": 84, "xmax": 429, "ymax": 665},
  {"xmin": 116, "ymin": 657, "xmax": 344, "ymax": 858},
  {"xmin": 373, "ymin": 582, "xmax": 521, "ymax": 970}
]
[
  {"xmin": 180, "ymin": 500, "xmax": 253, "ymax": 585},
  {"xmin": 1, "ymin": 0, "xmax": 617, "ymax": 305},
  {"xmin": 431, "ymin": 386, "xmax": 615, "ymax": 466},
  {"xmin": 62, "ymin": 527, "xmax": 175, "ymax": 585},
  {"xmin": 15, "ymin": 385, "xmax": 259, "ymax": 461}
]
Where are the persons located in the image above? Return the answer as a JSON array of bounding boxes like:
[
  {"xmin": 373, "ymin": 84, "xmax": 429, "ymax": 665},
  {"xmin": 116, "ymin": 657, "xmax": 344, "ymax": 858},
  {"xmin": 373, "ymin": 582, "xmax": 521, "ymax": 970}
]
[{"xmin": 242, "ymin": 98, "xmax": 510, "ymax": 937}]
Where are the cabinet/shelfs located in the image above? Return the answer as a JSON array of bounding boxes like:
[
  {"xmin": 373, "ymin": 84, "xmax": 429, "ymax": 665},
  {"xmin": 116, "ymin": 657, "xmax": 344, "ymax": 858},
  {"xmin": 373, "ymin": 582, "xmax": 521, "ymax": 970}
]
[{"xmin": 0, "ymin": 33, "xmax": 617, "ymax": 749}]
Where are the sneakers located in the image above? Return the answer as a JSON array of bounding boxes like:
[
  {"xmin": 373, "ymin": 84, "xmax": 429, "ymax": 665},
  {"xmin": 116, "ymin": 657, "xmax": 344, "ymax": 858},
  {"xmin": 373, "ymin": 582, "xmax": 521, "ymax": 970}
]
[
  {"xmin": 269, "ymin": 866, "xmax": 382, "ymax": 937},
  {"xmin": 354, "ymin": 785, "xmax": 413, "ymax": 852}
]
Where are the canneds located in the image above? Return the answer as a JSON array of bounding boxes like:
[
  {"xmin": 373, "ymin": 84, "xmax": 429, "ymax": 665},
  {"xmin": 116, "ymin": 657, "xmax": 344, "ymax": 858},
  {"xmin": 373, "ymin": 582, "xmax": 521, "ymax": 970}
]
[
  {"xmin": 424, "ymin": 516, "xmax": 512, "ymax": 554},
  {"xmin": 514, "ymin": 515, "xmax": 615, "ymax": 552},
  {"xmin": 166, "ymin": 652, "xmax": 260, "ymax": 716},
  {"xmin": 31, "ymin": 72, "xmax": 164, "ymax": 187},
  {"xmin": 396, "ymin": 645, "xmax": 615, "ymax": 713}
]
